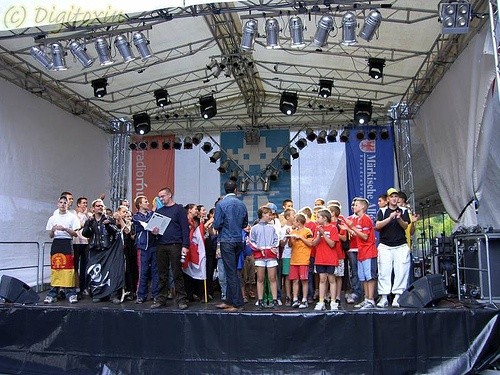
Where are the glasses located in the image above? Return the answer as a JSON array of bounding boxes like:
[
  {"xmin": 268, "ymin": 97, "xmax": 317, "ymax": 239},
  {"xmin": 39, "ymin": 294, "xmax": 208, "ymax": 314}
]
[
  {"xmin": 159, "ymin": 195, "xmax": 166, "ymax": 198},
  {"xmin": 96, "ymin": 205, "xmax": 104, "ymax": 207},
  {"xmin": 60, "ymin": 202, "xmax": 66, "ymax": 204}
]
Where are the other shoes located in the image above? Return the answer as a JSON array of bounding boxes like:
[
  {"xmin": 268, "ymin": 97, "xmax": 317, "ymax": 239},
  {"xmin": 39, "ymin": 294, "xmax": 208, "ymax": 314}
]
[
  {"xmin": 221, "ymin": 295, "xmax": 226, "ymax": 300},
  {"xmin": 179, "ymin": 300, "xmax": 188, "ymax": 310},
  {"xmin": 93, "ymin": 298, "xmax": 101, "ymax": 303},
  {"xmin": 60, "ymin": 289, "xmax": 65, "ymax": 293},
  {"xmin": 150, "ymin": 300, "xmax": 161, "ymax": 308},
  {"xmin": 126, "ymin": 291, "xmax": 134, "ymax": 300},
  {"xmin": 135, "ymin": 296, "xmax": 144, "ymax": 304},
  {"xmin": 69, "ymin": 295, "xmax": 78, "ymax": 304},
  {"xmin": 43, "ymin": 296, "xmax": 58, "ymax": 303},
  {"xmin": 249, "ymin": 291, "xmax": 257, "ymax": 298},
  {"xmin": 76, "ymin": 287, "xmax": 80, "ymax": 293},
  {"xmin": 112, "ymin": 297, "xmax": 121, "ymax": 304},
  {"xmin": 83, "ymin": 289, "xmax": 90, "ymax": 296},
  {"xmin": 215, "ymin": 303, "xmax": 245, "ymax": 311},
  {"xmin": 255, "ymin": 293, "xmax": 376, "ymax": 311},
  {"xmin": 243, "ymin": 296, "xmax": 248, "ymax": 303}
]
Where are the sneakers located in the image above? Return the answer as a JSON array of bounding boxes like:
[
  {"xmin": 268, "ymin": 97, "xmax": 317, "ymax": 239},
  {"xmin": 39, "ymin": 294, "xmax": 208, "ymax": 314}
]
[
  {"xmin": 376, "ymin": 298, "xmax": 388, "ymax": 308},
  {"xmin": 392, "ymin": 297, "xmax": 400, "ymax": 307}
]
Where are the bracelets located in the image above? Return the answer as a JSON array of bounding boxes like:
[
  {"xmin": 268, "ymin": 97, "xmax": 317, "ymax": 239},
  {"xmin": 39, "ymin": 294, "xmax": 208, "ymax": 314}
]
[{"xmin": 352, "ymin": 230, "xmax": 354, "ymax": 235}]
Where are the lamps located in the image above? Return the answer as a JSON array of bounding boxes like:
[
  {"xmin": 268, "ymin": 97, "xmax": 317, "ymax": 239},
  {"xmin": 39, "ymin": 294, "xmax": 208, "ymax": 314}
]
[
  {"xmin": 197, "ymin": 95, "xmax": 217, "ymax": 120},
  {"xmin": 353, "ymin": 99, "xmax": 373, "ymax": 124},
  {"xmin": 260, "ymin": 125, "xmax": 389, "ymax": 192},
  {"xmin": 319, "ymin": 79, "xmax": 334, "ymax": 99},
  {"xmin": 366, "ymin": 57, "xmax": 385, "ymax": 80},
  {"xmin": 238, "ymin": 9, "xmax": 383, "ymax": 51},
  {"xmin": 133, "ymin": 112, "xmax": 153, "ymax": 135},
  {"xmin": 90, "ymin": 77, "xmax": 107, "ymax": 98},
  {"xmin": 279, "ymin": 92, "xmax": 298, "ymax": 116},
  {"xmin": 437, "ymin": 0, "xmax": 472, "ymax": 34},
  {"xmin": 30, "ymin": 31, "xmax": 152, "ymax": 71},
  {"xmin": 207, "ymin": 55, "xmax": 259, "ymax": 79},
  {"xmin": 154, "ymin": 90, "xmax": 171, "ymax": 107},
  {"xmin": 125, "ymin": 126, "xmax": 255, "ymax": 192}
]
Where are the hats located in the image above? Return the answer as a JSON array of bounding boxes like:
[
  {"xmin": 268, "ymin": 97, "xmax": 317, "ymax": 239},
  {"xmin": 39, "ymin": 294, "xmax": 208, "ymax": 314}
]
[
  {"xmin": 387, "ymin": 188, "xmax": 398, "ymax": 196},
  {"xmin": 262, "ymin": 203, "xmax": 277, "ymax": 210}
]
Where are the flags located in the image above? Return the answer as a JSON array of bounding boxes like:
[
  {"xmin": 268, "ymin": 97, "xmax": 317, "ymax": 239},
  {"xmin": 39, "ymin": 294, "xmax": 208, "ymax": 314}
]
[
  {"xmin": 86, "ymin": 228, "xmax": 127, "ymax": 299},
  {"xmin": 180, "ymin": 222, "xmax": 206, "ymax": 279}
]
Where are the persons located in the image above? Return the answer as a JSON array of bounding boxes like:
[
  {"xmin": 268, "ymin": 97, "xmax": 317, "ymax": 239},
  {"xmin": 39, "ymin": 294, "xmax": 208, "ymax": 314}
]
[
  {"xmin": 212, "ymin": 177, "xmax": 248, "ymax": 312},
  {"xmin": 150, "ymin": 187, "xmax": 190, "ymax": 310},
  {"xmin": 45, "ymin": 192, "xmax": 420, "ymax": 311}
]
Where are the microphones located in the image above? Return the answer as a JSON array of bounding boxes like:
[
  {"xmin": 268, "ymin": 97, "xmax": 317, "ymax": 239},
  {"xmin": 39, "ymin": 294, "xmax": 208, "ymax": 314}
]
[
  {"xmin": 180, "ymin": 252, "xmax": 186, "ymax": 263},
  {"xmin": 475, "ymin": 201, "xmax": 478, "ymax": 214}
]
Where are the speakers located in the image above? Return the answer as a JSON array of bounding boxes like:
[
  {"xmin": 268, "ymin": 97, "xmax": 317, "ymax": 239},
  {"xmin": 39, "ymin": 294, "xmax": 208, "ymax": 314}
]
[
  {"xmin": 397, "ymin": 274, "xmax": 445, "ymax": 309},
  {"xmin": 0, "ymin": 275, "xmax": 40, "ymax": 305}
]
